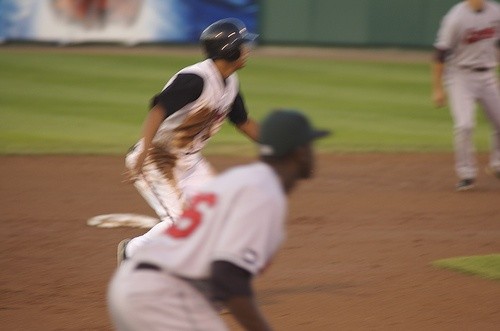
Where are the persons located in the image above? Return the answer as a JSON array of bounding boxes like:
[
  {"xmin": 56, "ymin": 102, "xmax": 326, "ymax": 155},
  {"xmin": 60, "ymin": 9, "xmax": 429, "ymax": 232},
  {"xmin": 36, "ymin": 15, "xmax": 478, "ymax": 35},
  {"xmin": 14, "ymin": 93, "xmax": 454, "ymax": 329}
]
[
  {"xmin": 116, "ymin": 22, "xmax": 263, "ymax": 267},
  {"xmin": 106, "ymin": 107, "xmax": 332, "ymax": 331},
  {"xmin": 427, "ymin": 0, "xmax": 500, "ymax": 192}
]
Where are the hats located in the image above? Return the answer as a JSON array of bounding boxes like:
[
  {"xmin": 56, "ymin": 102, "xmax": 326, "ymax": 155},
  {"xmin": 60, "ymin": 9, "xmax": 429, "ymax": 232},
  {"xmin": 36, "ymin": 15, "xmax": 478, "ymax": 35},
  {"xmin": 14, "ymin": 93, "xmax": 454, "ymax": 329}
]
[{"xmin": 255, "ymin": 109, "xmax": 328, "ymax": 161}]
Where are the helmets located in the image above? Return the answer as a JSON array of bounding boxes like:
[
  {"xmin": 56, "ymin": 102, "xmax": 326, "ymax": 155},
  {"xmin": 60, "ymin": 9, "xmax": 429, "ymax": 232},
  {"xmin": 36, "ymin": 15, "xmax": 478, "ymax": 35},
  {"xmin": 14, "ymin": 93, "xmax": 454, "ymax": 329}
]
[{"xmin": 200, "ymin": 17, "xmax": 259, "ymax": 57}]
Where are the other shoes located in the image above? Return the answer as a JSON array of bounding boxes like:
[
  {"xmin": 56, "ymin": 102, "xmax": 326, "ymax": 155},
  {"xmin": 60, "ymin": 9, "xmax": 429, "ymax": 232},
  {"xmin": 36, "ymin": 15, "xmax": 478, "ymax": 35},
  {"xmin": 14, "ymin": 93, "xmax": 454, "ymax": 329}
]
[
  {"xmin": 455, "ymin": 179, "xmax": 478, "ymax": 190},
  {"xmin": 117, "ymin": 237, "xmax": 130, "ymax": 265}
]
